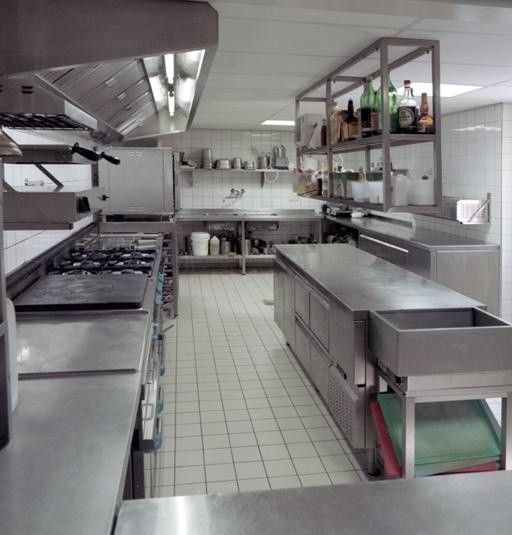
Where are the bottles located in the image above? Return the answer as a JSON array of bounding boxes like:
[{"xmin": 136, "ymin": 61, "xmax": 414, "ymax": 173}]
[
  {"xmin": 359, "ymin": 74, "xmax": 376, "ymax": 134},
  {"xmin": 340, "ymin": 98, "xmax": 360, "ymax": 140},
  {"xmin": 317, "ymin": 170, "xmax": 329, "ymax": 198},
  {"xmin": 357, "ymin": 167, "xmax": 366, "ymax": 183},
  {"xmin": 209, "ymin": 234, "xmax": 221, "ymax": 256},
  {"xmin": 398, "ymin": 79, "xmax": 420, "ymax": 132},
  {"xmin": 373, "ymin": 66, "xmax": 399, "ymax": 133},
  {"xmin": 415, "ymin": 93, "xmax": 435, "ymax": 132}
]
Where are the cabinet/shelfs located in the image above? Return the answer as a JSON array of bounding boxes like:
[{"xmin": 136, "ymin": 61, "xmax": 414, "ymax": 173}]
[
  {"xmin": 37, "ymin": 46, "xmax": 157, "ymax": 137},
  {"xmin": 272, "ymin": 251, "xmax": 363, "ymax": 451},
  {"xmin": 290, "ymin": 31, "xmax": 444, "ymax": 217},
  {"xmin": 359, "ymin": 231, "xmax": 500, "ymax": 311}
]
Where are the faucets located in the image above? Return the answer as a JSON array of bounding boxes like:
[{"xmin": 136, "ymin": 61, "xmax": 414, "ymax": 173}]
[{"xmin": 222, "ymin": 190, "xmax": 240, "ymax": 201}]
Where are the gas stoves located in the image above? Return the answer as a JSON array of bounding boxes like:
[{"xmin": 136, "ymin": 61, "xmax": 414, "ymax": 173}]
[{"xmin": 51, "ymin": 244, "xmax": 155, "ymax": 273}]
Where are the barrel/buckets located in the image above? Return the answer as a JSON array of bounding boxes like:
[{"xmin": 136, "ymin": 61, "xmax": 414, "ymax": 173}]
[
  {"xmin": 390, "ymin": 171, "xmax": 408, "ymax": 207},
  {"xmin": 408, "ymin": 173, "xmax": 434, "ymax": 207},
  {"xmin": 211, "ymin": 237, "xmax": 219, "ymax": 255},
  {"xmin": 190, "ymin": 231, "xmax": 210, "ymax": 255}
]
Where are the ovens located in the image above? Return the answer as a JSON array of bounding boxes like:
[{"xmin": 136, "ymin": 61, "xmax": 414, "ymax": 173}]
[{"xmin": 96, "ymin": 146, "xmax": 183, "ymax": 217}]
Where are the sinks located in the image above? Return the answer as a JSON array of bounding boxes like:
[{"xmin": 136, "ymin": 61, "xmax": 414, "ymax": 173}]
[
  {"xmin": 244, "ymin": 213, "xmax": 277, "ymax": 217},
  {"xmin": 203, "ymin": 213, "xmax": 238, "ymax": 217}
]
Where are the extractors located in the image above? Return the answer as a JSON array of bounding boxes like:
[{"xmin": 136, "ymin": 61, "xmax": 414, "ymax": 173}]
[{"xmin": 0, "ymin": 1, "xmax": 219, "ymax": 146}]
